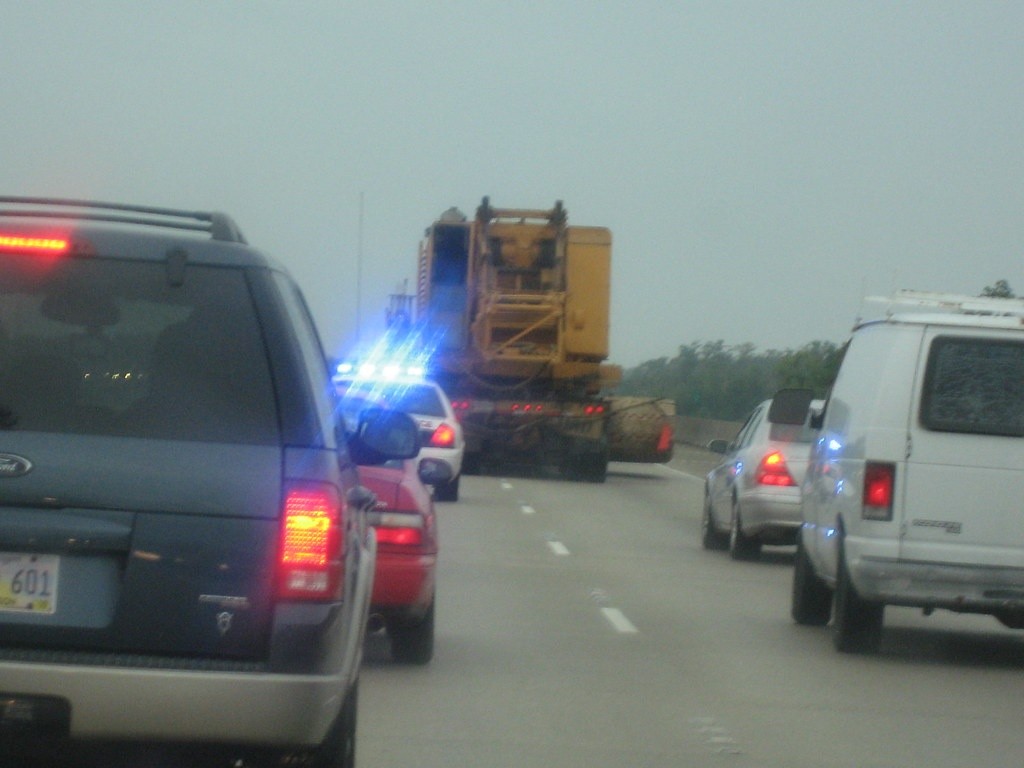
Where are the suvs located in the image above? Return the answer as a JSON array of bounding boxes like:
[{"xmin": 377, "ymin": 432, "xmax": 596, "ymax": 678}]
[{"xmin": 0, "ymin": 188, "xmax": 422, "ymax": 768}]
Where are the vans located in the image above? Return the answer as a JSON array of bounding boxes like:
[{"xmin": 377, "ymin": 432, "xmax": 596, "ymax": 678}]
[{"xmin": 764, "ymin": 289, "xmax": 1023, "ymax": 652}]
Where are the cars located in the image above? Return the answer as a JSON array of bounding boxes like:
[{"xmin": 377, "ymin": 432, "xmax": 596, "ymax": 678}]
[
  {"xmin": 329, "ymin": 379, "xmax": 457, "ymax": 672},
  {"xmin": 699, "ymin": 398, "xmax": 832, "ymax": 560},
  {"xmin": 327, "ymin": 374, "xmax": 465, "ymax": 500}
]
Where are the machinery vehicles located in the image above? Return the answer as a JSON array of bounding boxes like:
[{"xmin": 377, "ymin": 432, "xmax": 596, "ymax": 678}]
[{"xmin": 386, "ymin": 196, "xmax": 681, "ymax": 482}]
